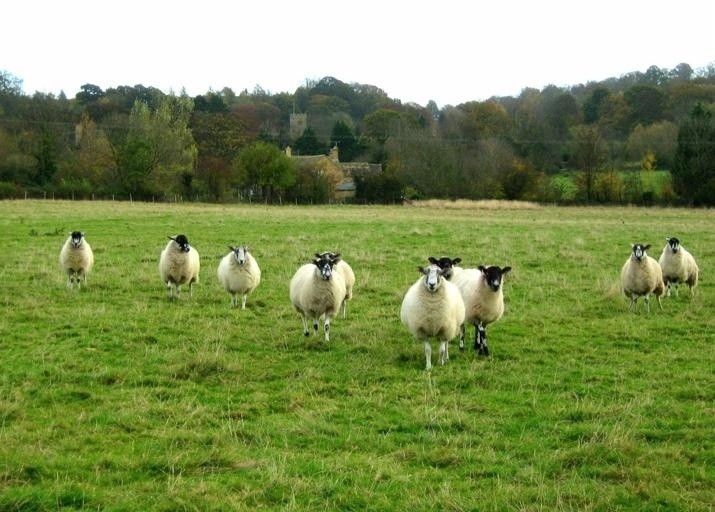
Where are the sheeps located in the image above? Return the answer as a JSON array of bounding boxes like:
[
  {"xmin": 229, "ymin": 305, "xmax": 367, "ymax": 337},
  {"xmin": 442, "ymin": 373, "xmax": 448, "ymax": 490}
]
[
  {"xmin": 289, "ymin": 251, "xmax": 355, "ymax": 342},
  {"xmin": 658, "ymin": 236, "xmax": 698, "ymax": 298},
  {"xmin": 159, "ymin": 234, "xmax": 200, "ymax": 300},
  {"xmin": 217, "ymin": 244, "xmax": 261, "ymax": 311},
  {"xmin": 60, "ymin": 230, "xmax": 94, "ymax": 290},
  {"xmin": 401, "ymin": 256, "xmax": 512, "ymax": 370},
  {"xmin": 620, "ymin": 242, "xmax": 665, "ymax": 312}
]
